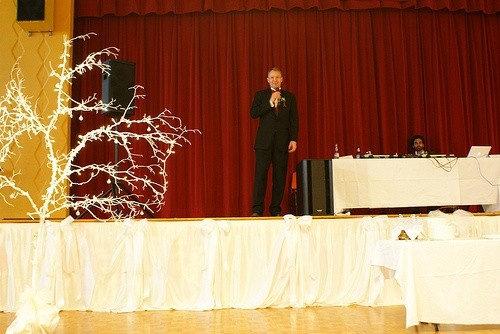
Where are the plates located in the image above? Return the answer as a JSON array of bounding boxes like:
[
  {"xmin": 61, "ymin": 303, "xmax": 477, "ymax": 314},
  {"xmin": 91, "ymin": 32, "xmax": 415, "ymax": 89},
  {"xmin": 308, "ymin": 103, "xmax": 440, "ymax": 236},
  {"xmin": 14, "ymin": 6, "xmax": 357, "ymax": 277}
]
[{"xmin": 373, "ymin": 155, "xmax": 390, "ymax": 158}]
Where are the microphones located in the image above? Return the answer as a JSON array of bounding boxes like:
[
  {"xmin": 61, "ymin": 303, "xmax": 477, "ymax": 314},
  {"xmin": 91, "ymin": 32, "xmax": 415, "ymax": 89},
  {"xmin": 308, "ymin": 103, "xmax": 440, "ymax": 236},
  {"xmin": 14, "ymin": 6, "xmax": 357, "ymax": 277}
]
[{"xmin": 275, "ymin": 87, "xmax": 281, "ymax": 106}]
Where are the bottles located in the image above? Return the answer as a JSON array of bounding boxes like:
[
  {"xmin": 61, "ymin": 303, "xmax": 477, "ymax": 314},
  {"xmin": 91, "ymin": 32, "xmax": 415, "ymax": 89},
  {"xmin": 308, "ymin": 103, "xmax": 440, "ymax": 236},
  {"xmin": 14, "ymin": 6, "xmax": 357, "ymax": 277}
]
[
  {"xmin": 334, "ymin": 144, "xmax": 340, "ymax": 159},
  {"xmin": 356, "ymin": 147, "xmax": 361, "ymax": 158}
]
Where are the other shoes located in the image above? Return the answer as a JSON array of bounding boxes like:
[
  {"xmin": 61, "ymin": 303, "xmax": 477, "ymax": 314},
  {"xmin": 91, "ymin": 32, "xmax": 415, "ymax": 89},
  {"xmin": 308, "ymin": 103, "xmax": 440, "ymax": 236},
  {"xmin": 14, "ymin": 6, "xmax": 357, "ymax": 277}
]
[
  {"xmin": 251, "ymin": 212, "xmax": 260, "ymax": 217},
  {"xmin": 271, "ymin": 212, "xmax": 281, "ymax": 216}
]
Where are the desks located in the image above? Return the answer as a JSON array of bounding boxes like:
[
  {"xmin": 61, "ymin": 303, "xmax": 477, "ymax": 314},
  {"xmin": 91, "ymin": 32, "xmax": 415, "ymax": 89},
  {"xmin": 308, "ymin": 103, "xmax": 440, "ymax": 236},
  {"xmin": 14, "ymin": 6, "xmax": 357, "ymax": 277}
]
[
  {"xmin": 367, "ymin": 241, "xmax": 500, "ymax": 331},
  {"xmin": 333, "ymin": 155, "xmax": 500, "ymax": 218}
]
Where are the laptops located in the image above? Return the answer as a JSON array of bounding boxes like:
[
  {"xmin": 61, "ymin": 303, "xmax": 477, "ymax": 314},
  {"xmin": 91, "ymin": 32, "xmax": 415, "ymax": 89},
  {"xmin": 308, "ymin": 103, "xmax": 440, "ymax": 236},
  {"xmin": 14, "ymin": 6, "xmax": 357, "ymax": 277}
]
[{"xmin": 467, "ymin": 146, "xmax": 492, "ymax": 158}]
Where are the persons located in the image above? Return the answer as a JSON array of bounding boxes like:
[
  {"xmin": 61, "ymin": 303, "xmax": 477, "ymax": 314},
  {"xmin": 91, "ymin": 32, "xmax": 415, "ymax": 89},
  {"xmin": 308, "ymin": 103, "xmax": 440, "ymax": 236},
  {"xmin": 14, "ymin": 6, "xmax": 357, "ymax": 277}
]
[
  {"xmin": 251, "ymin": 68, "xmax": 299, "ymax": 216},
  {"xmin": 411, "ymin": 135, "xmax": 433, "ymax": 157}
]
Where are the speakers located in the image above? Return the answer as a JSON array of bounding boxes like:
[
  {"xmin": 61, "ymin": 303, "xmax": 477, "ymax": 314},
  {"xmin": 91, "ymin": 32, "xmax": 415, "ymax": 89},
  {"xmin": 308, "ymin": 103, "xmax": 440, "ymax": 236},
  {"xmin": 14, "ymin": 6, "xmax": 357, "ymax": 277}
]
[
  {"xmin": 16, "ymin": 0, "xmax": 54, "ymax": 32},
  {"xmin": 101, "ymin": 58, "xmax": 136, "ymax": 116},
  {"xmin": 296, "ymin": 159, "xmax": 334, "ymax": 216}
]
